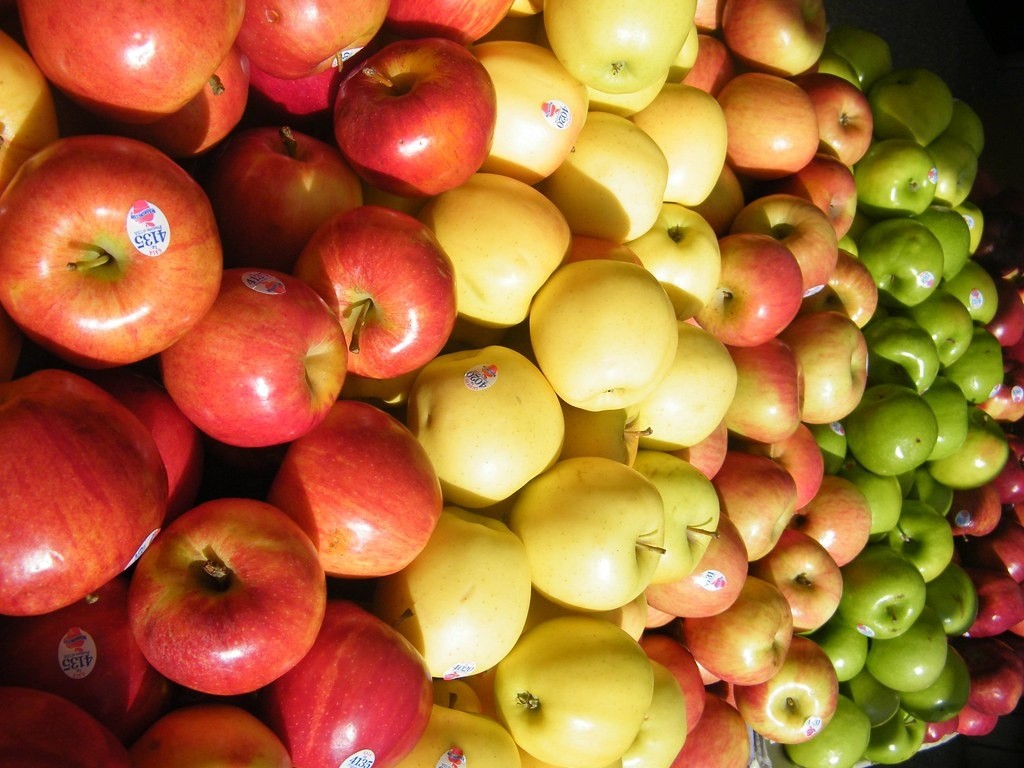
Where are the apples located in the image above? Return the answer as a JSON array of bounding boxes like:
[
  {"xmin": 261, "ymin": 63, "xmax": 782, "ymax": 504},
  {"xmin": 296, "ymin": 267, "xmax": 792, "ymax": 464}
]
[{"xmin": 0, "ymin": 0, "xmax": 1024, "ymax": 768}]
